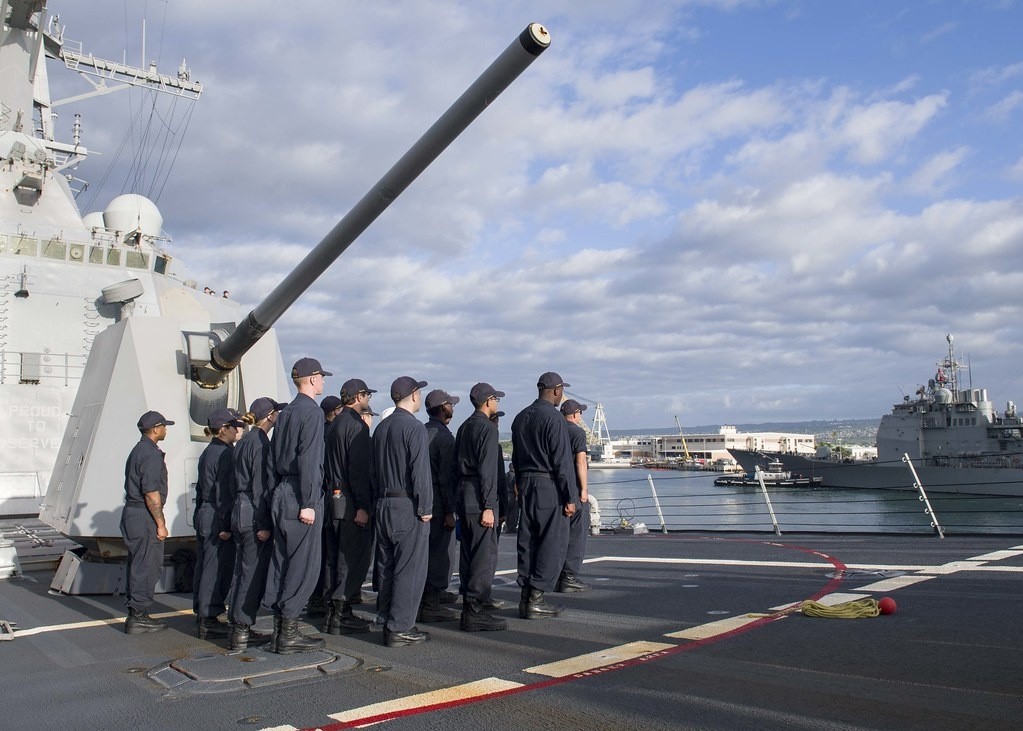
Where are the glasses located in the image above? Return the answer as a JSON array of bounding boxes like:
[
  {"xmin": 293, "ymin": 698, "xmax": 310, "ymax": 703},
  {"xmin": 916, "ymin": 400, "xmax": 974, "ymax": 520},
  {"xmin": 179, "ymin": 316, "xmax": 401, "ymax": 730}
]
[
  {"xmin": 363, "ymin": 393, "xmax": 373, "ymax": 398},
  {"xmin": 575, "ymin": 409, "xmax": 582, "ymax": 415},
  {"xmin": 492, "ymin": 398, "xmax": 500, "ymax": 402}
]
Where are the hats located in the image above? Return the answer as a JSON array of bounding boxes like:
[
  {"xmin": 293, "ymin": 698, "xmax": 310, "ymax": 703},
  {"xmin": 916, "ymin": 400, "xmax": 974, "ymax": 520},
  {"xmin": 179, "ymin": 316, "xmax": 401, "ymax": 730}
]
[
  {"xmin": 538, "ymin": 371, "xmax": 570, "ymax": 388},
  {"xmin": 470, "ymin": 382, "xmax": 505, "ymax": 405},
  {"xmin": 291, "ymin": 358, "xmax": 332, "ymax": 378},
  {"xmin": 137, "ymin": 411, "xmax": 174, "ymax": 429},
  {"xmin": 360, "ymin": 406, "xmax": 380, "ymax": 417},
  {"xmin": 340, "ymin": 378, "xmax": 376, "ymax": 396},
  {"xmin": 321, "ymin": 395, "xmax": 342, "ymax": 413},
  {"xmin": 425, "ymin": 390, "xmax": 459, "ymax": 410},
  {"xmin": 491, "ymin": 411, "xmax": 504, "ymax": 420},
  {"xmin": 391, "ymin": 376, "xmax": 429, "ymax": 401},
  {"xmin": 560, "ymin": 399, "xmax": 587, "ymax": 414},
  {"xmin": 251, "ymin": 397, "xmax": 289, "ymax": 420},
  {"xmin": 206, "ymin": 408, "xmax": 245, "ymax": 430}
]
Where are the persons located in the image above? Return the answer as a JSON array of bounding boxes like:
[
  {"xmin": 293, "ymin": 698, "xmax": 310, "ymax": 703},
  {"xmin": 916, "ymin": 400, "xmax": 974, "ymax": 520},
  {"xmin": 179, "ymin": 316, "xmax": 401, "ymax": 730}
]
[
  {"xmin": 453, "ymin": 382, "xmax": 509, "ymax": 630},
  {"xmin": 193, "ymin": 395, "xmax": 506, "ymax": 639},
  {"xmin": 119, "ymin": 411, "xmax": 175, "ymax": 633},
  {"xmin": 324, "ymin": 379, "xmax": 376, "ymax": 636},
  {"xmin": 508, "ymin": 461, "xmax": 519, "ymax": 533},
  {"xmin": 509, "ymin": 371, "xmax": 580, "ymax": 619},
  {"xmin": 266, "ymin": 357, "xmax": 327, "ymax": 654},
  {"xmin": 370, "ymin": 378, "xmax": 431, "ymax": 648},
  {"xmin": 553, "ymin": 397, "xmax": 593, "ymax": 594}
]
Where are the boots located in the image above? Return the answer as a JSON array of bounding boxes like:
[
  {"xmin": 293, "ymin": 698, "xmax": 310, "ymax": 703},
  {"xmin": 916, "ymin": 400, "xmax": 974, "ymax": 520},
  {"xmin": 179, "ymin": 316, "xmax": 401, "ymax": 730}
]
[
  {"xmin": 519, "ymin": 586, "xmax": 565, "ymax": 619},
  {"xmin": 195, "ymin": 589, "xmax": 501, "ymax": 654},
  {"xmin": 554, "ymin": 571, "xmax": 591, "ymax": 592},
  {"xmin": 123, "ymin": 607, "xmax": 169, "ymax": 634}
]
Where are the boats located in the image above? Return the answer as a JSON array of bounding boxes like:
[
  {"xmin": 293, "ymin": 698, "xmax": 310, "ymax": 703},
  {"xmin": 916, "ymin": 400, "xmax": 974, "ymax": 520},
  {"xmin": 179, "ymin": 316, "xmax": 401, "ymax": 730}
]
[
  {"xmin": 714, "ymin": 458, "xmax": 823, "ymax": 487},
  {"xmin": 727, "ymin": 331, "xmax": 1023, "ymax": 496}
]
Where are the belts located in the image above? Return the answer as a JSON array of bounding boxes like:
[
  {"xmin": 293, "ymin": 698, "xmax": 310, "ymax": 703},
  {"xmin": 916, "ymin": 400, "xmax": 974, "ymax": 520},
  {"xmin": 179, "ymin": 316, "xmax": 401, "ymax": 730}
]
[
  {"xmin": 521, "ymin": 472, "xmax": 555, "ymax": 479},
  {"xmin": 379, "ymin": 492, "xmax": 413, "ymax": 497}
]
